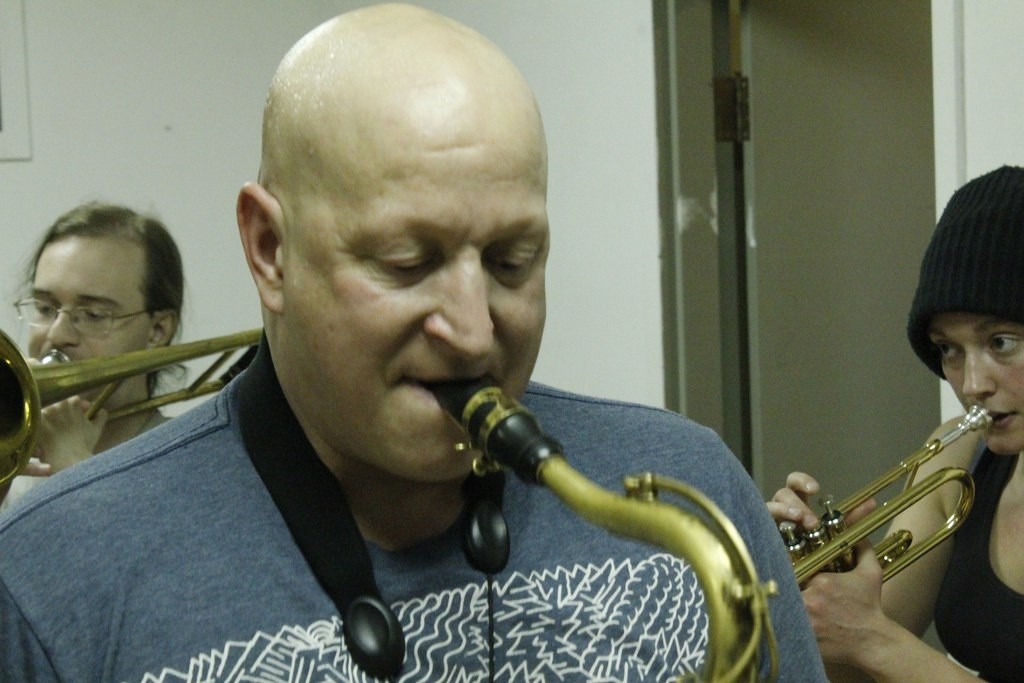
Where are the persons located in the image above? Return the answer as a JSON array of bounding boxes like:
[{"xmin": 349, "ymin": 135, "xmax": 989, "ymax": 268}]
[
  {"xmin": 763, "ymin": 163, "xmax": 1024, "ymax": 683},
  {"xmin": 1, "ymin": 202, "xmax": 183, "ymax": 512},
  {"xmin": 0, "ymin": 0, "xmax": 828, "ymax": 683}
]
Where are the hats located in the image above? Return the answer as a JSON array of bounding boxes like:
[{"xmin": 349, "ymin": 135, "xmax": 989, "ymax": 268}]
[{"xmin": 907, "ymin": 165, "xmax": 1024, "ymax": 381}]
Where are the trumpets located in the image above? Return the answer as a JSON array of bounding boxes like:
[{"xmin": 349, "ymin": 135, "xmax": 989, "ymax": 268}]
[{"xmin": 778, "ymin": 404, "xmax": 994, "ymax": 593}]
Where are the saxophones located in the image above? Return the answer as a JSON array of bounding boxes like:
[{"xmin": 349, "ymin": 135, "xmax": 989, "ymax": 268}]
[{"xmin": 439, "ymin": 374, "xmax": 781, "ymax": 683}]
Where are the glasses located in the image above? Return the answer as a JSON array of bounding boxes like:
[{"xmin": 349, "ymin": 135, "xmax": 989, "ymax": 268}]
[{"xmin": 14, "ymin": 295, "xmax": 150, "ymax": 338}]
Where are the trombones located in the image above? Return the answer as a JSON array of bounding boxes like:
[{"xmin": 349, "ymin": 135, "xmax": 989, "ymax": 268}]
[{"xmin": 0, "ymin": 325, "xmax": 264, "ymax": 485}]
